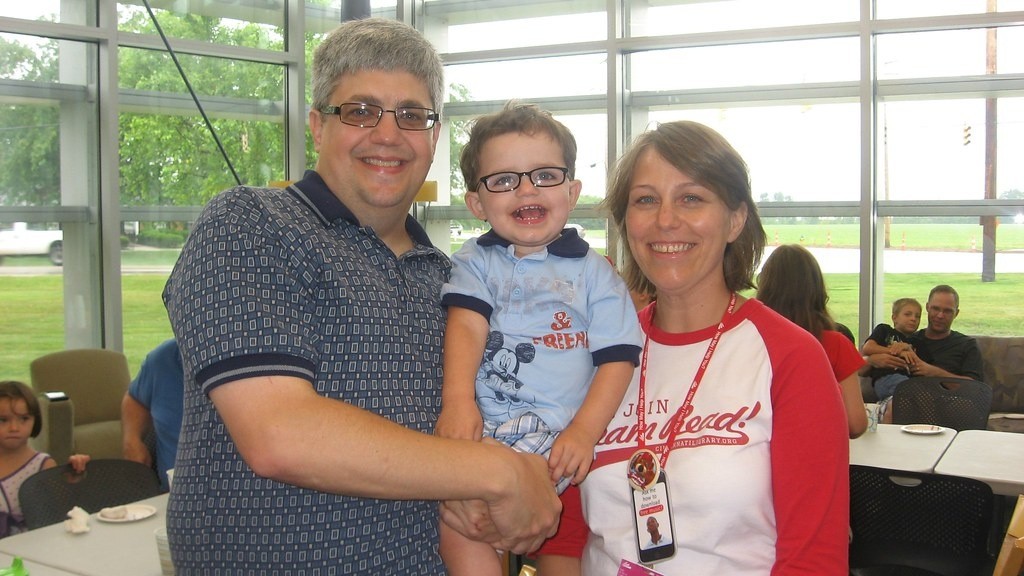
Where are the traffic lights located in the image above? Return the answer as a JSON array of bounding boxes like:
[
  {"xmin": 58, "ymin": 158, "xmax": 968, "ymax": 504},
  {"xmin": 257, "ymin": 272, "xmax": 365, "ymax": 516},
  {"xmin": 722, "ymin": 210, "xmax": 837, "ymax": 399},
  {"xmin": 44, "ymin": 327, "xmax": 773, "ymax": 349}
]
[{"xmin": 964, "ymin": 124, "xmax": 971, "ymax": 146}]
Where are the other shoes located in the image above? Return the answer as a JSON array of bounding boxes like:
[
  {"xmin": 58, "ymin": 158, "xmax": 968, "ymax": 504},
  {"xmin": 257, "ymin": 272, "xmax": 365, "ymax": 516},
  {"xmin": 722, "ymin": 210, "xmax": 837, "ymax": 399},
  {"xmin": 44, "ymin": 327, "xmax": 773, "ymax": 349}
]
[{"xmin": 889, "ymin": 475, "xmax": 921, "ymax": 486}]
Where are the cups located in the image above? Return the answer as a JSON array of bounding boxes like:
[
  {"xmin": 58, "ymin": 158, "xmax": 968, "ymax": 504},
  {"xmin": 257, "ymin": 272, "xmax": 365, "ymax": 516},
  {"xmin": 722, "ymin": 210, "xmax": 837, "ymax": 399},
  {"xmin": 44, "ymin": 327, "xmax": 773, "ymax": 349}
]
[
  {"xmin": 864, "ymin": 403, "xmax": 880, "ymax": 433},
  {"xmin": 153, "ymin": 527, "xmax": 176, "ymax": 576},
  {"xmin": 166, "ymin": 469, "xmax": 174, "ymax": 490}
]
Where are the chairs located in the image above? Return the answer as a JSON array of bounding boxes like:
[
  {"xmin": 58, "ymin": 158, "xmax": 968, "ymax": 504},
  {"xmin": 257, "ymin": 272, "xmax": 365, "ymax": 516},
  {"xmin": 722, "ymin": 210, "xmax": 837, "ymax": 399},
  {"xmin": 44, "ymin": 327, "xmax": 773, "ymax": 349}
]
[
  {"xmin": 848, "ymin": 464, "xmax": 995, "ymax": 576},
  {"xmin": 893, "ymin": 375, "xmax": 994, "ymax": 431},
  {"xmin": 26, "ymin": 349, "xmax": 135, "ymax": 461},
  {"xmin": 18, "ymin": 460, "xmax": 163, "ymax": 531}
]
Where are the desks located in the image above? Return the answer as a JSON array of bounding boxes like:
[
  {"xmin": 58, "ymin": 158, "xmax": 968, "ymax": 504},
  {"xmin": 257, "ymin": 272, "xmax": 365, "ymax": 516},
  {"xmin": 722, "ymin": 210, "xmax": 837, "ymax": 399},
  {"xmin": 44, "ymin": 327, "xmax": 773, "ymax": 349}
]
[
  {"xmin": 847, "ymin": 422, "xmax": 1024, "ymax": 496},
  {"xmin": 1, "ymin": 493, "xmax": 176, "ymax": 576}
]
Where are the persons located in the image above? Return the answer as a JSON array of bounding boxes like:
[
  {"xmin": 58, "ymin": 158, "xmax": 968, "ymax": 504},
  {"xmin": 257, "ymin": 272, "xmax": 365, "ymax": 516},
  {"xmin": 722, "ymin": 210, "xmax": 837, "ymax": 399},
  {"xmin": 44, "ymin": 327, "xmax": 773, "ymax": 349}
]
[
  {"xmin": 121, "ymin": 337, "xmax": 184, "ymax": 495},
  {"xmin": 432, "ymin": 98, "xmax": 645, "ymax": 576},
  {"xmin": 162, "ymin": 16, "xmax": 562, "ymax": 576},
  {"xmin": 526, "ymin": 122, "xmax": 849, "ymax": 576},
  {"xmin": 0, "ymin": 381, "xmax": 91, "ymax": 537},
  {"xmin": 882, "ymin": 284, "xmax": 985, "ymax": 423},
  {"xmin": 861, "ymin": 297, "xmax": 922, "ymax": 401},
  {"xmin": 756, "ymin": 244, "xmax": 869, "ymax": 440}
]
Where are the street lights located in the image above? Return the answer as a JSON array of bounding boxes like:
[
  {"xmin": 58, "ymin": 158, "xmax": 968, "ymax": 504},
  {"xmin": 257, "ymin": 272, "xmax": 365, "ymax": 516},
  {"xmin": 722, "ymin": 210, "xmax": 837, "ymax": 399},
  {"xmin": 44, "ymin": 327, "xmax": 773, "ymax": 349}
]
[{"xmin": 880, "ymin": 59, "xmax": 898, "ymax": 247}]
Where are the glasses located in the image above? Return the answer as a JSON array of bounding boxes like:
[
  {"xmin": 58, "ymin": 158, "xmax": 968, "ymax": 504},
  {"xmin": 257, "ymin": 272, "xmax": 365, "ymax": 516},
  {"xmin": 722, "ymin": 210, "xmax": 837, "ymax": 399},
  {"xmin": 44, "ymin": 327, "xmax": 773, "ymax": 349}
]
[
  {"xmin": 475, "ymin": 166, "xmax": 568, "ymax": 193},
  {"xmin": 320, "ymin": 102, "xmax": 439, "ymax": 130}
]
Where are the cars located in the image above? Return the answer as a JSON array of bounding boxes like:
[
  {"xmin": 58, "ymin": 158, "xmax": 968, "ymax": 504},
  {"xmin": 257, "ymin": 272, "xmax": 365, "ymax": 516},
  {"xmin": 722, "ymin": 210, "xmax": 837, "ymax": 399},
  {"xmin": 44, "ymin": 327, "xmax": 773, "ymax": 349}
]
[
  {"xmin": 0, "ymin": 220, "xmax": 64, "ymax": 268},
  {"xmin": 563, "ymin": 222, "xmax": 586, "ymax": 238}
]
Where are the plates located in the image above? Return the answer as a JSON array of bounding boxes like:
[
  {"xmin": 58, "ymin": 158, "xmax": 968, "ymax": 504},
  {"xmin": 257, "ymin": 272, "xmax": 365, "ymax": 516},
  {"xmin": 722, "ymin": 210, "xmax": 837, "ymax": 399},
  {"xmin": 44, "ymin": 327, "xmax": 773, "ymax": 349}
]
[
  {"xmin": 97, "ymin": 504, "xmax": 156, "ymax": 522},
  {"xmin": 901, "ymin": 424, "xmax": 944, "ymax": 434}
]
